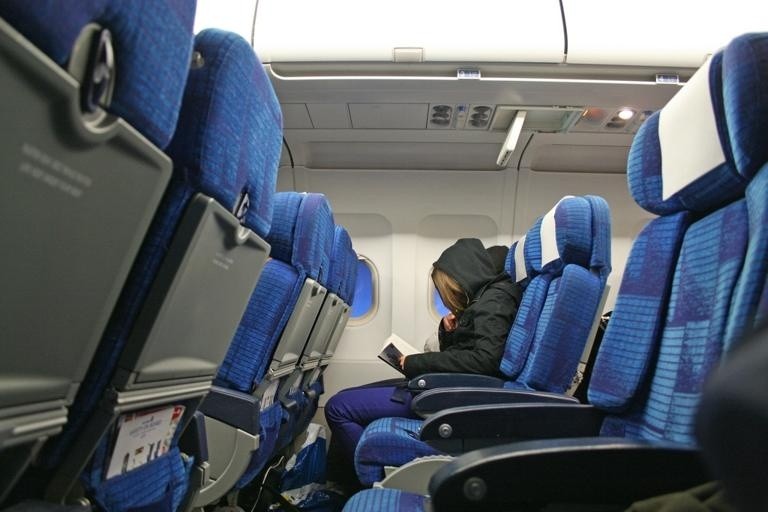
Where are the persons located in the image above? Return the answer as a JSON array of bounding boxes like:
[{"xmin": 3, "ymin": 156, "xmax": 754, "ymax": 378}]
[
  {"xmin": 325, "ymin": 238, "xmax": 523, "ymax": 460},
  {"xmin": 327, "ymin": 247, "xmax": 507, "ymax": 480}
]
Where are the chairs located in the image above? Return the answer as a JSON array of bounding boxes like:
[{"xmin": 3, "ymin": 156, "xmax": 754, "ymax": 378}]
[
  {"xmin": 50, "ymin": 24, "xmax": 289, "ymax": 509},
  {"xmin": 4, "ymin": 3, "xmax": 199, "ymax": 509},
  {"xmin": 405, "ymin": 190, "xmax": 612, "ymax": 416},
  {"xmin": 234, "ymin": 228, "xmax": 366, "ymax": 487},
  {"xmin": 353, "ymin": 104, "xmax": 685, "ymax": 487},
  {"xmin": 344, "ymin": 31, "xmax": 768, "ymax": 511},
  {"xmin": 178, "ymin": 188, "xmax": 336, "ymax": 508}
]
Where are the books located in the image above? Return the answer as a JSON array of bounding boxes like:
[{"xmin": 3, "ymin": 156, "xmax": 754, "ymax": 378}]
[{"xmin": 378, "ymin": 334, "xmax": 419, "ymax": 377}]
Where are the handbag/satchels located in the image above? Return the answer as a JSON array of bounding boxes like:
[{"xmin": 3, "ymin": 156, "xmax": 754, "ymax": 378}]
[
  {"xmin": 238, "ymin": 455, "xmax": 302, "ymax": 511},
  {"xmin": 280, "ymin": 423, "xmax": 331, "ymax": 509}
]
